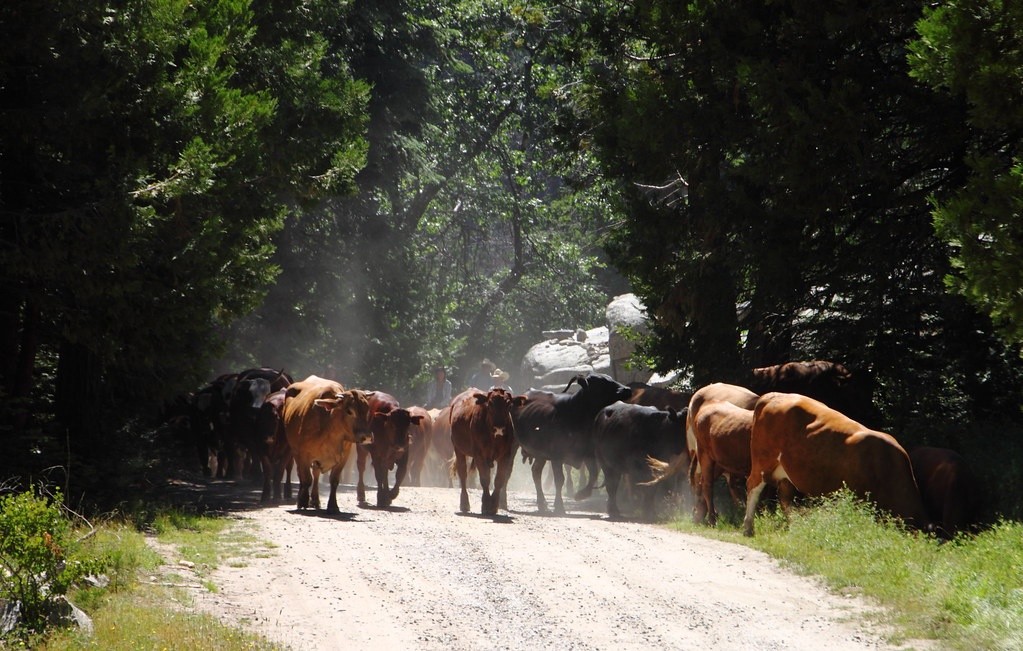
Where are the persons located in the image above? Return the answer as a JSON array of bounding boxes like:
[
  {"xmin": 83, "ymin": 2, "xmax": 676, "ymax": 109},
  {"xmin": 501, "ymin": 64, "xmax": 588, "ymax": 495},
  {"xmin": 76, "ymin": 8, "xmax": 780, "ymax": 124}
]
[
  {"xmin": 422, "ymin": 363, "xmax": 452, "ymax": 410},
  {"xmin": 471, "ymin": 358, "xmax": 513, "ymax": 395}
]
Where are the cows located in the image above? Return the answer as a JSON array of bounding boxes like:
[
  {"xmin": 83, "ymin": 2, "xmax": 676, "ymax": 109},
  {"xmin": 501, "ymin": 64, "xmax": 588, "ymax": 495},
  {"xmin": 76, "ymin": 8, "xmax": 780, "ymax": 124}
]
[{"xmin": 180, "ymin": 360, "xmax": 977, "ymax": 532}]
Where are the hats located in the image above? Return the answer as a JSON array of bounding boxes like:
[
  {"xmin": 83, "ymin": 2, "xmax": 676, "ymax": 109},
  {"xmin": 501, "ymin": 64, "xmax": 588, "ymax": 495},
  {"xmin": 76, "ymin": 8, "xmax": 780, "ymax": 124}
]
[
  {"xmin": 480, "ymin": 358, "xmax": 496, "ymax": 371},
  {"xmin": 489, "ymin": 368, "xmax": 509, "ymax": 382}
]
